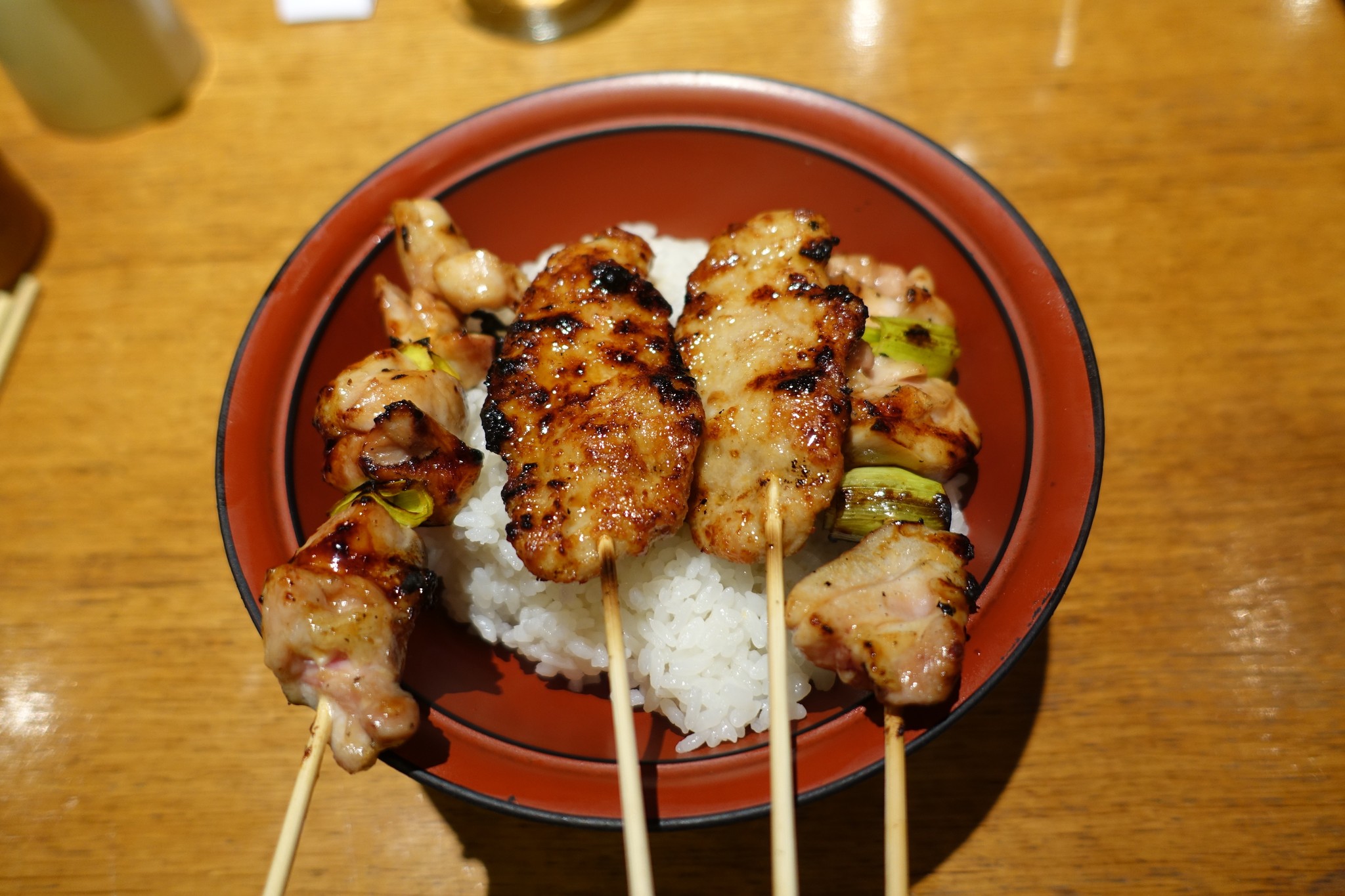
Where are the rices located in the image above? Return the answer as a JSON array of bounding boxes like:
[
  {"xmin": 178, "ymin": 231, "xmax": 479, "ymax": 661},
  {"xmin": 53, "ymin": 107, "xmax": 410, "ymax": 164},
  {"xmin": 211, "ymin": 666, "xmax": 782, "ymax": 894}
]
[{"xmin": 405, "ymin": 213, "xmax": 968, "ymax": 751}]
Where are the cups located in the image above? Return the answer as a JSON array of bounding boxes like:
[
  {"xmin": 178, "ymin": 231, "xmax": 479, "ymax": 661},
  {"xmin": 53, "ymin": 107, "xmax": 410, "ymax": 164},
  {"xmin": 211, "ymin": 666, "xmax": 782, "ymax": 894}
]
[{"xmin": 1, "ymin": 0, "xmax": 201, "ymax": 133}]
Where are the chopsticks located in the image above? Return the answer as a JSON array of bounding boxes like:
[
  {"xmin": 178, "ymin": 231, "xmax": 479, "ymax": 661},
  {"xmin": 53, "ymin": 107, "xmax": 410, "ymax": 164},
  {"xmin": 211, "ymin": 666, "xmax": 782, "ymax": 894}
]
[{"xmin": 0, "ymin": 272, "xmax": 40, "ymax": 384}]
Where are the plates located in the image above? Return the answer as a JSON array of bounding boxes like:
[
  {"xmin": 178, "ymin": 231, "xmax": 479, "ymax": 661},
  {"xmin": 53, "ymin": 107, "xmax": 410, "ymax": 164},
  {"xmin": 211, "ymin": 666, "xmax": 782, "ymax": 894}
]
[{"xmin": 211, "ymin": 70, "xmax": 1106, "ymax": 830}]
